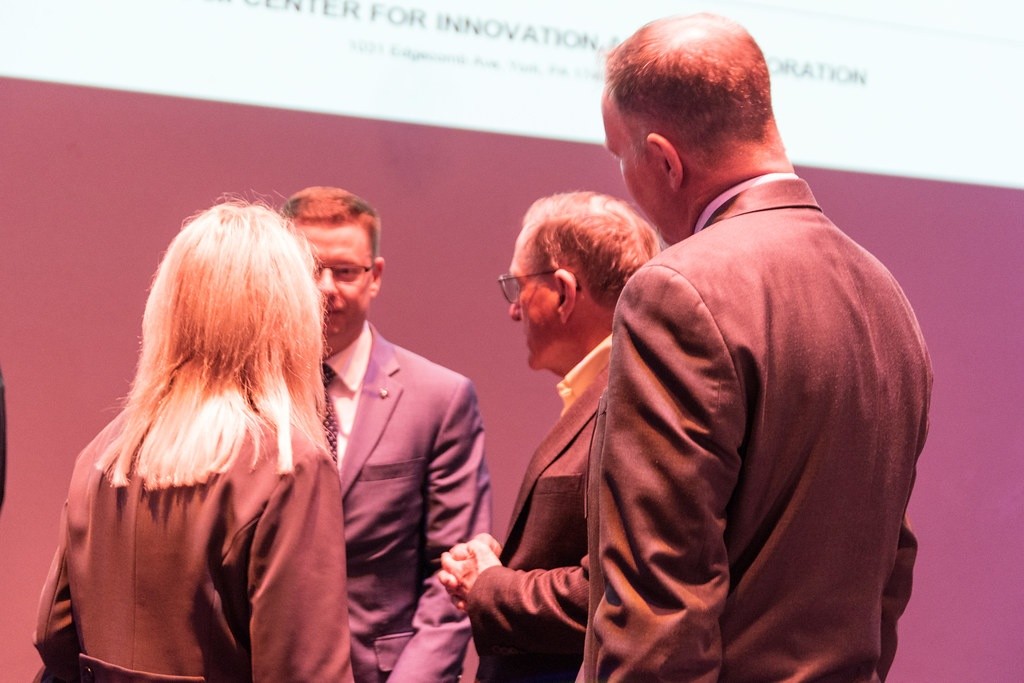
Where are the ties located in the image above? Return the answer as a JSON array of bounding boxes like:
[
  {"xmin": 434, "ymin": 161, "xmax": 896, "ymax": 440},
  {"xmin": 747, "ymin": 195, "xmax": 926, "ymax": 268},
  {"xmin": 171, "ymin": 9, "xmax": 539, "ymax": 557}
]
[{"xmin": 322, "ymin": 363, "xmax": 338, "ymax": 466}]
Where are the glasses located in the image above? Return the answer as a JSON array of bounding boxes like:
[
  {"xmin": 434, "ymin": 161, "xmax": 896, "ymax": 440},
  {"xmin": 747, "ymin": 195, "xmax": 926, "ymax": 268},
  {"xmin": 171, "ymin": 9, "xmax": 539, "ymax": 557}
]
[
  {"xmin": 497, "ymin": 270, "xmax": 556, "ymax": 303},
  {"xmin": 319, "ymin": 264, "xmax": 373, "ymax": 282}
]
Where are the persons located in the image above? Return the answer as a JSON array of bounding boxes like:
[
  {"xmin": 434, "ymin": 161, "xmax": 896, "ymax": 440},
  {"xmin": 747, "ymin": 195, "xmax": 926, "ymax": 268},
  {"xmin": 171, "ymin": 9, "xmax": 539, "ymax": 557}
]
[
  {"xmin": 571, "ymin": 13, "xmax": 932, "ymax": 683},
  {"xmin": 285, "ymin": 189, "xmax": 493, "ymax": 683},
  {"xmin": 32, "ymin": 199, "xmax": 353, "ymax": 683},
  {"xmin": 437, "ymin": 190, "xmax": 661, "ymax": 683}
]
